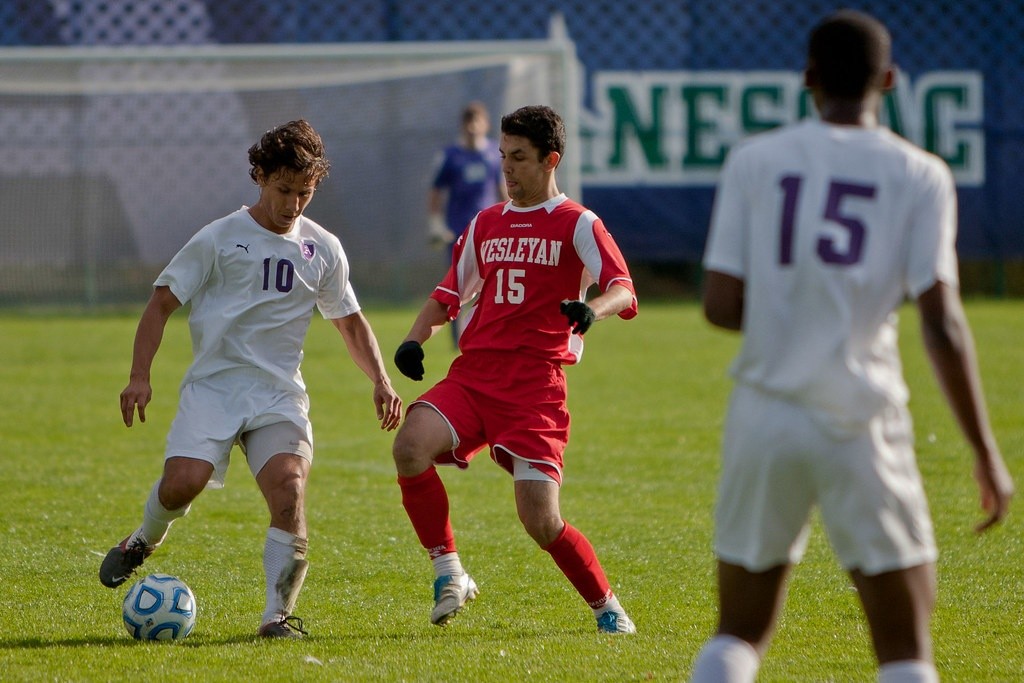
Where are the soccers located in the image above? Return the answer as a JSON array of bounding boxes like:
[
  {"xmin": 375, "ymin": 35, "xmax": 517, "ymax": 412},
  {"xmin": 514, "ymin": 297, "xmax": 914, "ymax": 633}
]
[{"xmin": 123, "ymin": 569, "xmax": 198, "ymax": 643}]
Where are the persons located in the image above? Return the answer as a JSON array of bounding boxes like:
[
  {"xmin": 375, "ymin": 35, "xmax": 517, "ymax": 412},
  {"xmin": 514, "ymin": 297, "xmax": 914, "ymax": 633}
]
[
  {"xmin": 690, "ymin": 10, "xmax": 1012, "ymax": 683},
  {"xmin": 99, "ymin": 119, "xmax": 402, "ymax": 636},
  {"xmin": 391, "ymin": 106, "xmax": 640, "ymax": 634},
  {"xmin": 421, "ymin": 102, "xmax": 513, "ymax": 349}
]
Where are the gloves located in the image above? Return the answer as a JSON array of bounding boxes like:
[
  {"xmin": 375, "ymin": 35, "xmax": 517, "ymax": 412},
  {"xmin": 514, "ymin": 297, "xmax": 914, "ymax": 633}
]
[
  {"xmin": 560, "ymin": 299, "xmax": 595, "ymax": 335},
  {"xmin": 394, "ymin": 341, "xmax": 424, "ymax": 381},
  {"xmin": 428, "ymin": 216, "xmax": 455, "ymax": 245}
]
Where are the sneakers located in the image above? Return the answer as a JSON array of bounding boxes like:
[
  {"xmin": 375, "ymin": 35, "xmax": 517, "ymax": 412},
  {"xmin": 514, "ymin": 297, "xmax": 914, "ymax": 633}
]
[
  {"xmin": 596, "ymin": 610, "xmax": 636, "ymax": 636},
  {"xmin": 431, "ymin": 568, "xmax": 480, "ymax": 628},
  {"xmin": 258, "ymin": 608, "xmax": 308, "ymax": 640},
  {"xmin": 99, "ymin": 523, "xmax": 163, "ymax": 589}
]
